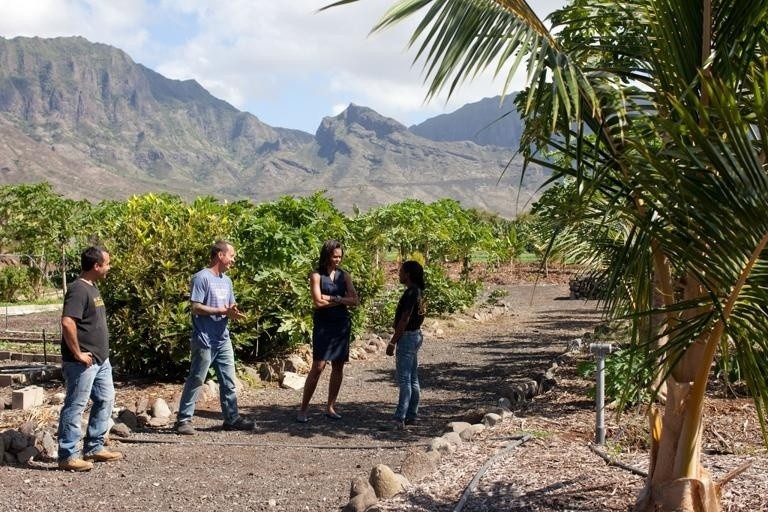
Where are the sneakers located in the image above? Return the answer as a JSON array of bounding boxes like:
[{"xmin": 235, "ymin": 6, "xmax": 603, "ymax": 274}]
[
  {"xmin": 58, "ymin": 458, "xmax": 93, "ymax": 471},
  {"xmin": 405, "ymin": 417, "xmax": 417, "ymax": 425},
  {"xmin": 84, "ymin": 449, "xmax": 123, "ymax": 463},
  {"xmin": 223, "ymin": 416, "xmax": 257, "ymax": 430},
  {"xmin": 379, "ymin": 418, "xmax": 405, "ymax": 431},
  {"xmin": 176, "ymin": 420, "xmax": 195, "ymax": 434}
]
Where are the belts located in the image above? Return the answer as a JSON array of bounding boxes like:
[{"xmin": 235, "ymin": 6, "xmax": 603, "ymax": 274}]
[{"xmin": 402, "ymin": 328, "xmax": 421, "ymax": 336}]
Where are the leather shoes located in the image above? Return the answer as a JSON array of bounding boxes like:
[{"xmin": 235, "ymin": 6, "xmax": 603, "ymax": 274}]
[
  {"xmin": 329, "ymin": 412, "xmax": 341, "ymax": 418},
  {"xmin": 296, "ymin": 414, "xmax": 307, "ymax": 422}
]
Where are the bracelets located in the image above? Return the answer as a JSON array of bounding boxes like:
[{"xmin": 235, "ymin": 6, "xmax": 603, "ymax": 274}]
[
  {"xmin": 334, "ymin": 294, "xmax": 342, "ymax": 302},
  {"xmin": 389, "ymin": 342, "xmax": 395, "ymax": 347}
]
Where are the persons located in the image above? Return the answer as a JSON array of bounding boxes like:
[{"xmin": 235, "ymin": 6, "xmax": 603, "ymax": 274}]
[
  {"xmin": 295, "ymin": 238, "xmax": 359, "ymax": 423},
  {"xmin": 172, "ymin": 241, "xmax": 258, "ymax": 436},
  {"xmin": 54, "ymin": 246, "xmax": 126, "ymax": 471},
  {"xmin": 385, "ymin": 259, "xmax": 426, "ymax": 425}
]
